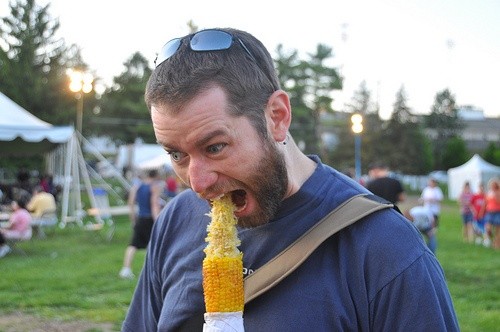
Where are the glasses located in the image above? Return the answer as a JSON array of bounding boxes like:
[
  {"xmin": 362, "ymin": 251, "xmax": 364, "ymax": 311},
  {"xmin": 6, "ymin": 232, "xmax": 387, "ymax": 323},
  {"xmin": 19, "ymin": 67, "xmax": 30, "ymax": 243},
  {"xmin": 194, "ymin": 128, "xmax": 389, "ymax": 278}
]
[{"xmin": 154, "ymin": 29, "xmax": 277, "ymax": 91}]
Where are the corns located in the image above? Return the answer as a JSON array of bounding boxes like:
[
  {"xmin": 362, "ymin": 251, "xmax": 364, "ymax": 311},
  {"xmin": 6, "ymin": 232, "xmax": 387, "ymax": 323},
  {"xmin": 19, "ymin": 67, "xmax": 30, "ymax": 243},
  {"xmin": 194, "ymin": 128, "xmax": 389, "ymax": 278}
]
[{"xmin": 202, "ymin": 191, "xmax": 245, "ymax": 313}]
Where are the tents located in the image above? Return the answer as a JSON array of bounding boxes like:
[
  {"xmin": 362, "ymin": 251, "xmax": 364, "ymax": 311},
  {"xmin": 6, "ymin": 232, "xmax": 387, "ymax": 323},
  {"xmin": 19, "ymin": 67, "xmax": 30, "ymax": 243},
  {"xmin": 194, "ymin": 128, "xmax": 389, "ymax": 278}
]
[
  {"xmin": 0, "ymin": 93, "xmax": 75, "ymax": 228},
  {"xmin": 448, "ymin": 152, "xmax": 500, "ymax": 201}
]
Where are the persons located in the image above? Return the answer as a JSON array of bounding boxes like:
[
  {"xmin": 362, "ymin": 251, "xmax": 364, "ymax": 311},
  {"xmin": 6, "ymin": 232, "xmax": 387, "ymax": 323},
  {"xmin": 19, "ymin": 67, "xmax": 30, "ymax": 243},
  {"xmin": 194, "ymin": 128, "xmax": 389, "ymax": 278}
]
[
  {"xmin": 460, "ymin": 179, "xmax": 500, "ymax": 247},
  {"xmin": 26, "ymin": 186, "xmax": 56, "ymax": 239},
  {"xmin": 0, "ymin": 199, "xmax": 33, "ymax": 257},
  {"xmin": 402, "ymin": 206, "xmax": 437, "ymax": 254},
  {"xmin": 364, "ymin": 160, "xmax": 405, "ymax": 203},
  {"xmin": 166, "ymin": 174, "xmax": 178, "ymax": 197},
  {"xmin": 120, "ymin": 28, "xmax": 461, "ymax": 332},
  {"xmin": 417, "ymin": 178, "xmax": 443, "ymax": 226},
  {"xmin": 118, "ymin": 168, "xmax": 160, "ymax": 279}
]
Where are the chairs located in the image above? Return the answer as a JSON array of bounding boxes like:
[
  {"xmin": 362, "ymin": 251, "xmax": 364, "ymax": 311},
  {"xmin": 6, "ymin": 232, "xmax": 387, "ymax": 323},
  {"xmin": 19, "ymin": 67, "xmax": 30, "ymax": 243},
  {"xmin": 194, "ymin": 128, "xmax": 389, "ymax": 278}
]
[{"xmin": 3, "ymin": 207, "xmax": 59, "ymax": 260}]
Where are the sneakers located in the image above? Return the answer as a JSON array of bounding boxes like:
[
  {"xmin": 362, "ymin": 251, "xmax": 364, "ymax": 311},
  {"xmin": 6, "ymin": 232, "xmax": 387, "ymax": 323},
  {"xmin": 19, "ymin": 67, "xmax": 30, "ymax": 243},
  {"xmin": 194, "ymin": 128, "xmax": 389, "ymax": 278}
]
[
  {"xmin": 0, "ymin": 244, "xmax": 11, "ymax": 258},
  {"xmin": 119, "ymin": 268, "xmax": 136, "ymax": 279}
]
